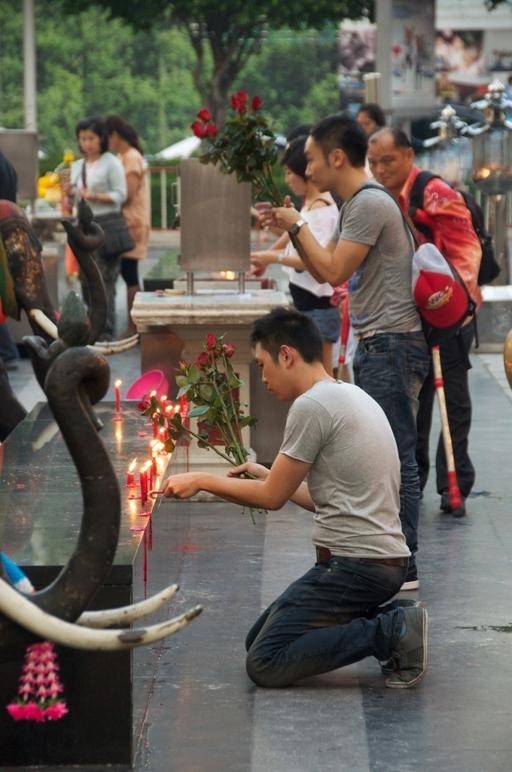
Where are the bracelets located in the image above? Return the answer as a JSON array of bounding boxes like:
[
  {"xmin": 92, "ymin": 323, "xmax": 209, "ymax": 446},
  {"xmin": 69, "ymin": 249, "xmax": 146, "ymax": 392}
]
[
  {"xmin": 93, "ymin": 191, "xmax": 100, "ymax": 203},
  {"xmin": 286, "ymin": 218, "xmax": 308, "ymax": 235}
]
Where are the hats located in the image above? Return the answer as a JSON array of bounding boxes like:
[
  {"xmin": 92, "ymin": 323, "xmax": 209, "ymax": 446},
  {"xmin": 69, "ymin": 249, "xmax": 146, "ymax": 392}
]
[{"xmin": 412, "ymin": 244, "xmax": 469, "ymax": 329}]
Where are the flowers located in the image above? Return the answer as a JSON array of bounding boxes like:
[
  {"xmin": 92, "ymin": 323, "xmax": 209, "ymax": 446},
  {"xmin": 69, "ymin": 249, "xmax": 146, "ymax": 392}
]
[
  {"xmin": 190, "ymin": 83, "xmax": 307, "ymax": 237},
  {"xmin": 131, "ymin": 329, "xmax": 276, "ymax": 548}
]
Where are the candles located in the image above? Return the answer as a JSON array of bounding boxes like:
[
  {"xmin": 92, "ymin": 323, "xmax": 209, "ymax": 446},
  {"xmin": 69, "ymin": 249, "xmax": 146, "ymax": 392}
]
[{"xmin": 108, "ymin": 367, "xmax": 180, "ymax": 527}]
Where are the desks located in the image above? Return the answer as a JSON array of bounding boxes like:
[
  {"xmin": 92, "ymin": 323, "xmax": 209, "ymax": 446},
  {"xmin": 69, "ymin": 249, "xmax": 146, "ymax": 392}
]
[{"xmin": 131, "ymin": 270, "xmax": 290, "ymax": 465}]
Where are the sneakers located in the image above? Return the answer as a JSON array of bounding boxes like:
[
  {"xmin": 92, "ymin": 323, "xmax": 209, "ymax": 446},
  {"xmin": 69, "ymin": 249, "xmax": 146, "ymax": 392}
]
[
  {"xmin": 440, "ymin": 491, "xmax": 468, "ymax": 519},
  {"xmin": 399, "ymin": 555, "xmax": 423, "ymax": 593},
  {"xmin": 385, "ymin": 606, "xmax": 429, "ymax": 691}
]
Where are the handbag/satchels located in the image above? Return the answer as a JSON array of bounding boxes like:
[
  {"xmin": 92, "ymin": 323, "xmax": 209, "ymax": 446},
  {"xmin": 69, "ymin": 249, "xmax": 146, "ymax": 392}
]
[
  {"xmin": 92, "ymin": 211, "xmax": 135, "ymax": 261},
  {"xmin": 351, "ymin": 186, "xmax": 480, "ymax": 352}
]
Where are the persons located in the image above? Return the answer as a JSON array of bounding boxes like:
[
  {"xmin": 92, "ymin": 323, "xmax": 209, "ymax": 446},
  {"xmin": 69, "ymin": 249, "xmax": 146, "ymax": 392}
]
[
  {"xmin": 68, "ymin": 116, "xmax": 128, "ymax": 346},
  {"xmin": 105, "ymin": 115, "xmax": 153, "ymax": 341},
  {"xmin": 157, "ymin": 304, "xmax": 428, "ymax": 689},
  {"xmin": 244, "ymin": 93, "xmax": 483, "ymax": 521},
  {"xmin": 253, "ymin": 111, "xmax": 435, "ymax": 593},
  {"xmin": 0, "ymin": 152, "xmax": 31, "ymax": 447}
]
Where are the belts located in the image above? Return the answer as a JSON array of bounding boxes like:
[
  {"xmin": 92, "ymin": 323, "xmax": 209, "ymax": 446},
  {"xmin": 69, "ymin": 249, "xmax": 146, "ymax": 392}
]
[{"xmin": 315, "ymin": 545, "xmax": 407, "ymax": 567}]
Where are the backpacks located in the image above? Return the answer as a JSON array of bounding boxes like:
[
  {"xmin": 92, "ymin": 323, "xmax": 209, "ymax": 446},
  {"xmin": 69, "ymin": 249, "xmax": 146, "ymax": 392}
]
[{"xmin": 407, "ymin": 170, "xmax": 499, "ymax": 288}]
